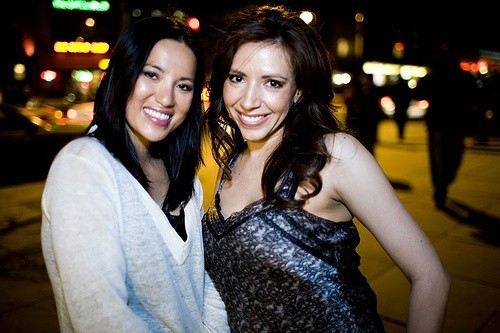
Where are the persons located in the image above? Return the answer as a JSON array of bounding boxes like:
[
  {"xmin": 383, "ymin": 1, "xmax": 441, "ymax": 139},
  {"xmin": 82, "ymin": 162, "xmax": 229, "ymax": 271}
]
[
  {"xmin": 201, "ymin": 3, "xmax": 452, "ymax": 333},
  {"xmin": 37, "ymin": 11, "xmax": 233, "ymax": 332},
  {"xmin": 343, "ymin": 39, "xmax": 499, "ymax": 211}
]
